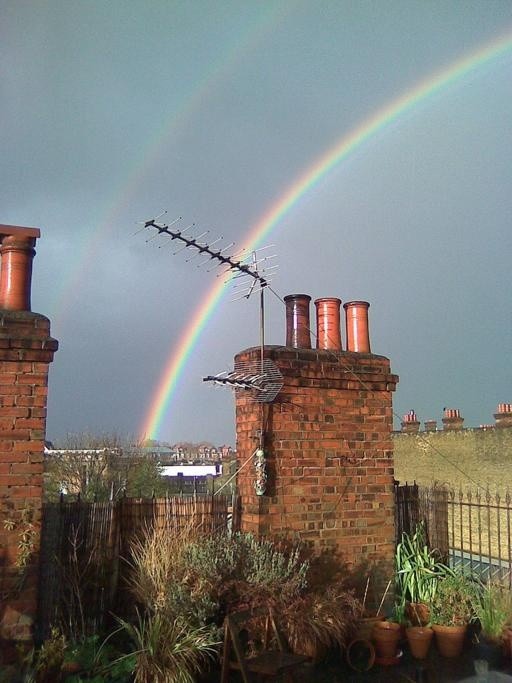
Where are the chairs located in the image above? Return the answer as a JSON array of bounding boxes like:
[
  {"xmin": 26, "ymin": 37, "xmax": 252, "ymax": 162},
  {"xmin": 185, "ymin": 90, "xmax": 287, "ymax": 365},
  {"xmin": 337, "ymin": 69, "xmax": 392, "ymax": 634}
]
[{"xmin": 219, "ymin": 602, "xmax": 313, "ymax": 683}]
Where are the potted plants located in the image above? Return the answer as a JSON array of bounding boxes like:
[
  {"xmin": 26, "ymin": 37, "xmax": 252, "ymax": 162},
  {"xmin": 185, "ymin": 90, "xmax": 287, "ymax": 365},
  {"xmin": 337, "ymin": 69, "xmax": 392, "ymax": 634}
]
[{"xmin": 393, "ymin": 519, "xmax": 512, "ymax": 658}]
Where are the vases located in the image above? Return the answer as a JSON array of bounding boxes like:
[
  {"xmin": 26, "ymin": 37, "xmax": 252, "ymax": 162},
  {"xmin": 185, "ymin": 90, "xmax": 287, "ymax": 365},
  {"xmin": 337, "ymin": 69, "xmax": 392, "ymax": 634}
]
[{"xmin": 341, "ymin": 613, "xmax": 400, "ymax": 673}]
